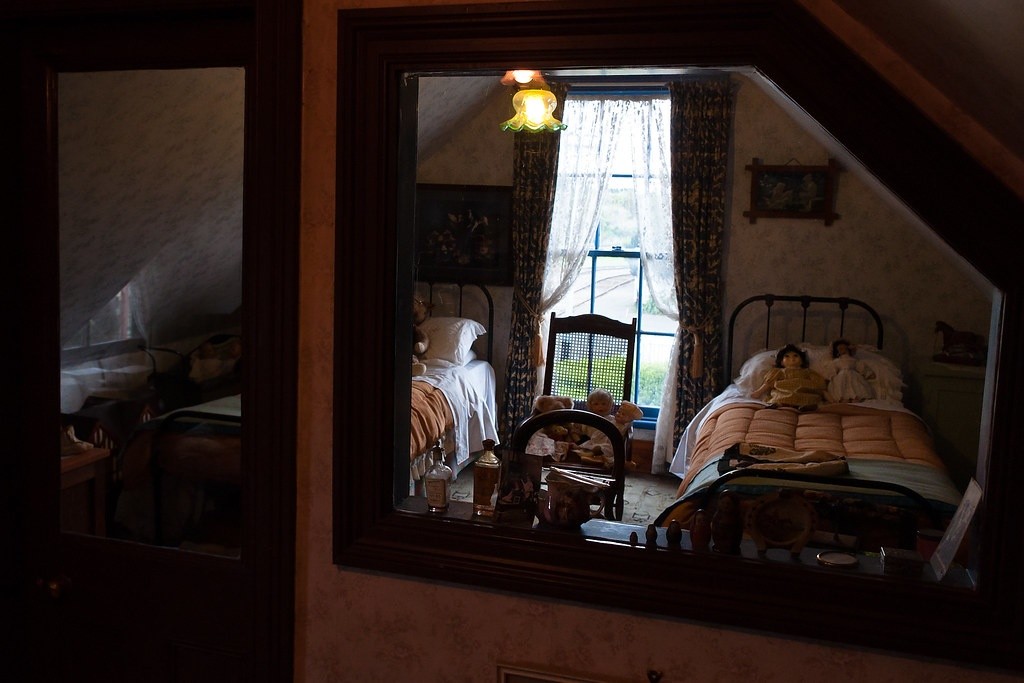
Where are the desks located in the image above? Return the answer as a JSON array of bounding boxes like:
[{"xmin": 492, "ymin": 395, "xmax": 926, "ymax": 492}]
[
  {"xmin": 911, "ymin": 362, "xmax": 985, "ymax": 473},
  {"xmin": 60, "ymin": 448, "xmax": 110, "ymax": 539}
]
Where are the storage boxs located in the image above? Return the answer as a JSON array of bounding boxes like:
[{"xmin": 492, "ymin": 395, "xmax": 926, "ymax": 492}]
[{"xmin": 880, "ymin": 547, "xmax": 924, "ymax": 575}]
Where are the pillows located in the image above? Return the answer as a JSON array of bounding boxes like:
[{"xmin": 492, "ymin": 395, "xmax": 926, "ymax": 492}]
[
  {"xmin": 417, "ymin": 318, "xmax": 488, "ymax": 364},
  {"xmin": 732, "ymin": 344, "xmax": 907, "ymax": 401}
]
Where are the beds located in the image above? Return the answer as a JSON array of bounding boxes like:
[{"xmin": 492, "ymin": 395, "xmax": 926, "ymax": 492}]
[
  {"xmin": 118, "ymin": 277, "xmax": 501, "ymax": 543},
  {"xmin": 654, "ymin": 294, "xmax": 976, "ymax": 572}
]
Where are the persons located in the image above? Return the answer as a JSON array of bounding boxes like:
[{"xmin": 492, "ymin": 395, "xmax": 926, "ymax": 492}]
[
  {"xmin": 749, "ymin": 340, "xmax": 876, "ymax": 412},
  {"xmin": 575, "ymin": 389, "xmax": 644, "ymax": 469}
]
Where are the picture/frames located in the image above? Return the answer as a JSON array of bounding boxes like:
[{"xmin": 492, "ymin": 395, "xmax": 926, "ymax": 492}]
[{"xmin": 414, "ymin": 182, "xmax": 514, "ymax": 287}]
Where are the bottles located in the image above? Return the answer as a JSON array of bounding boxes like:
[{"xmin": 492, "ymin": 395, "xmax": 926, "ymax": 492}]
[
  {"xmin": 473, "ymin": 439, "xmax": 501, "ymax": 517},
  {"xmin": 424, "ymin": 446, "xmax": 453, "ymax": 512}
]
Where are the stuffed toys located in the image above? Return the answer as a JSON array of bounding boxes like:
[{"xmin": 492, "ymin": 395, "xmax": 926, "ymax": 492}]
[
  {"xmin": 413, "ymin": 294, "xmax": 430, "ymax": 376},
  {"xmin": 516, "ymin": 388, "xmax": 580, "ymax": 460}
]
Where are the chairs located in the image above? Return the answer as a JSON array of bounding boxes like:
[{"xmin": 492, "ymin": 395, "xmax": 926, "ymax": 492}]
[
  {"xmin": 542, "ymin": 313, "xmax": 636, "ymax": 522},
  {"xmin": 511, "ymin": 410, "xmax": 626, "ymax": 522}
]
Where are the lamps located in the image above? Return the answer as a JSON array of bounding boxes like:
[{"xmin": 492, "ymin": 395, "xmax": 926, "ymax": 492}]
[
  {"xmin": 500, "ymin": 70, "xmax": 551, "ymax": 90},
  {"xmin": 499, "ymin": 90, "xmax": 568, "ymax": 133}
]
